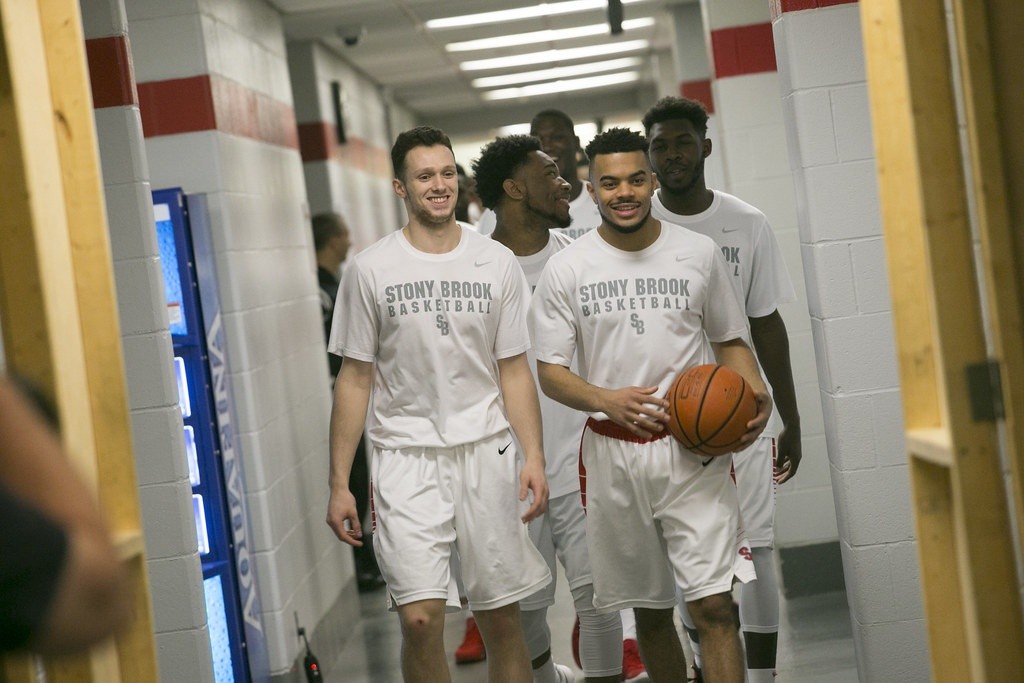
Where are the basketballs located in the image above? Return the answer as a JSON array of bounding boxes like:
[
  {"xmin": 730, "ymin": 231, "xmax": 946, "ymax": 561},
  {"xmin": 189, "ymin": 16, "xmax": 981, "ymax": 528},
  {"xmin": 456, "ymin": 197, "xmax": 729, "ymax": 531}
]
[{"xmin": 663, "ymin": 363, "xmax": 760, "ymax": 457}]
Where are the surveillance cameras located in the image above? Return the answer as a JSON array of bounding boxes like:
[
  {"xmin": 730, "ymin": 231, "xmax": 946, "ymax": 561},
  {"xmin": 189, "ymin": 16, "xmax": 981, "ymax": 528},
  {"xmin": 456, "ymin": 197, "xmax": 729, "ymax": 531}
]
[{"xmin": 337, "ymin": 25, "xmax": 362, "ymax": 45}]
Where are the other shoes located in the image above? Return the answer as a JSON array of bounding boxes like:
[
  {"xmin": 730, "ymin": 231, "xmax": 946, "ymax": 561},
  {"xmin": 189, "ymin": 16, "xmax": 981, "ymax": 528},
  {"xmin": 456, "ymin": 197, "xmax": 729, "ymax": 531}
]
[
  {"xmin": 548, "ymin": 663, "xmax": 576, "ymax": 683},
  {"xmin": 691, "ymin": 659, "xmax": 702, "ymax": 683}
]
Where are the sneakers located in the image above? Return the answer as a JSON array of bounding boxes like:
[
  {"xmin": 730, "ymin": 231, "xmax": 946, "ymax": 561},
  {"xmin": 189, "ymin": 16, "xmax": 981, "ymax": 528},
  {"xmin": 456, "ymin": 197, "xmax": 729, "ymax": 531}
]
[
  {"xmin": 620, "ymin": 638, "xmax": 648, "ymax": 683},
  {"xmin": 454, "ymin": 616, "xmax": 486, "ymax": 664}
]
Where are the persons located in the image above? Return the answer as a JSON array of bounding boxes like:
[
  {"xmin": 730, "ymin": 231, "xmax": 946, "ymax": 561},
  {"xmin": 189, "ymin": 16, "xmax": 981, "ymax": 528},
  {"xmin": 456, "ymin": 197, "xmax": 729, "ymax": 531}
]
[
  {"xmin": 0, "ymin": 373, "xmax": 120, "ymax": 683},
  {"xmin": 526, "ymin": 128, "xmax": 774, "ymax": 683},
  {"xmin": 313, "ymin": 212, "xmax": 386, "ymax": 594},
  {"xmin": 326, "ymin": 128, "xmax": 553, "ymax": 683},
  {"xmin": 644, "ymin": 97, "xmax": 804, "ymax": 683},
  {"xmin": 439, "ymin": 111, "xmax": 658, "ymax": 683}
]
